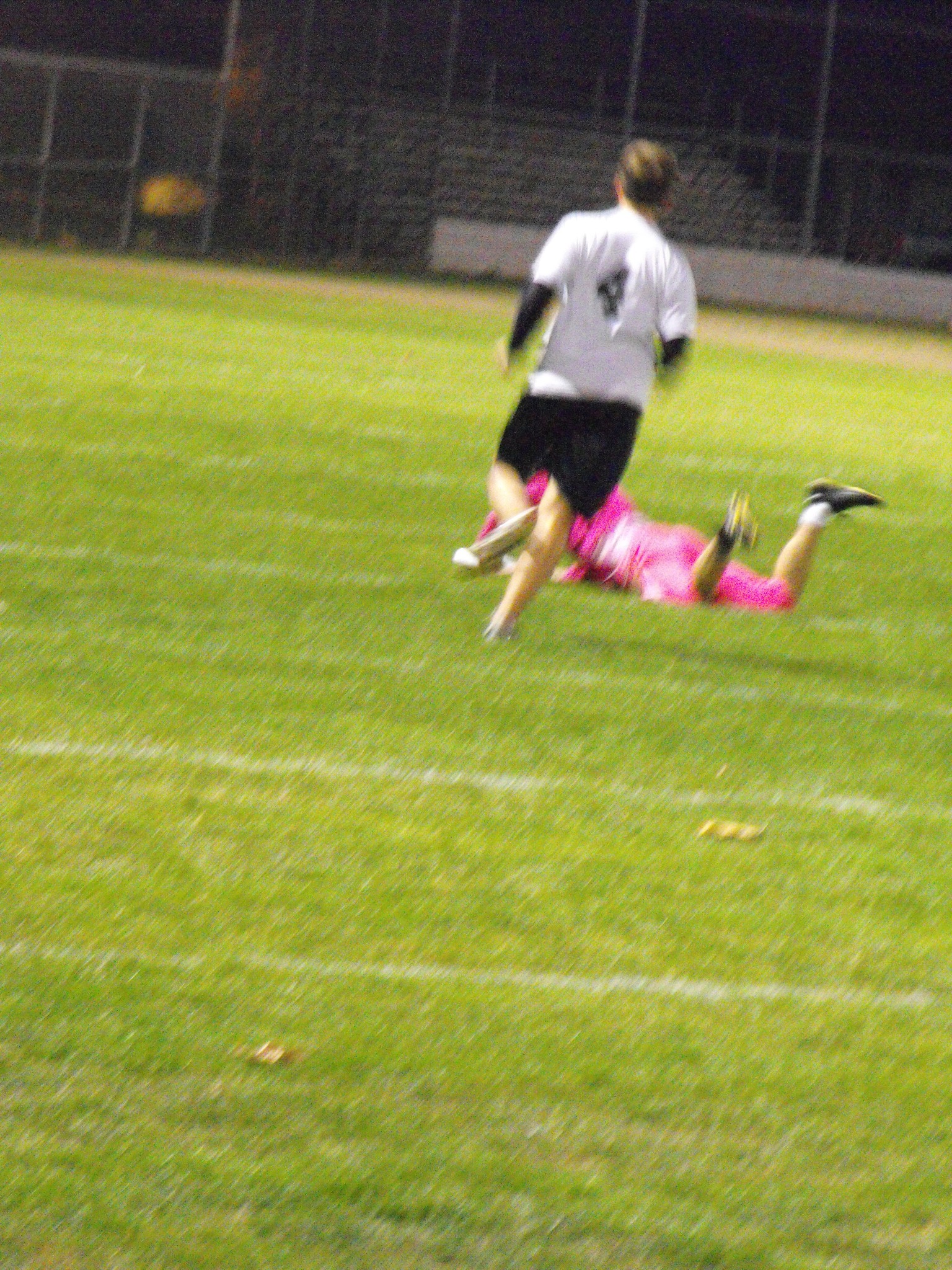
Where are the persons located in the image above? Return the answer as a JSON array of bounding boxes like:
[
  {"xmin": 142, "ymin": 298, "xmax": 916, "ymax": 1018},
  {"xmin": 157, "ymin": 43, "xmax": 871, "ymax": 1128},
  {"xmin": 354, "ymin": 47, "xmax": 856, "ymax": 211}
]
[
  {"xmin": 470, "ymin": 138, "xmax": 696, "ymax": 646},
  {"xmin": 453, "ymin": 470, "xmax": 888, "ymax": 615}
]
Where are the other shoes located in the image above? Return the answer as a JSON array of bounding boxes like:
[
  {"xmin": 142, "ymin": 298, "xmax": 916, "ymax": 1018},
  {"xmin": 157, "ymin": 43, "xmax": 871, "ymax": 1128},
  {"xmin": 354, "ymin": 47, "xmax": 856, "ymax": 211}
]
[
  {"xmin": 727, "ymin": 489, "xmax": 759, "ymax": 555},
  {"xmin": 483, "ymin": 623, "xmax": 513, "ymax": 643},
  {"xmin": 803, "ymin": 478, "xmax": 886, "ymax": 517},
  {"xmin": 451, "ymin": 506, "xmax": 539, "ymax": 571}
]
